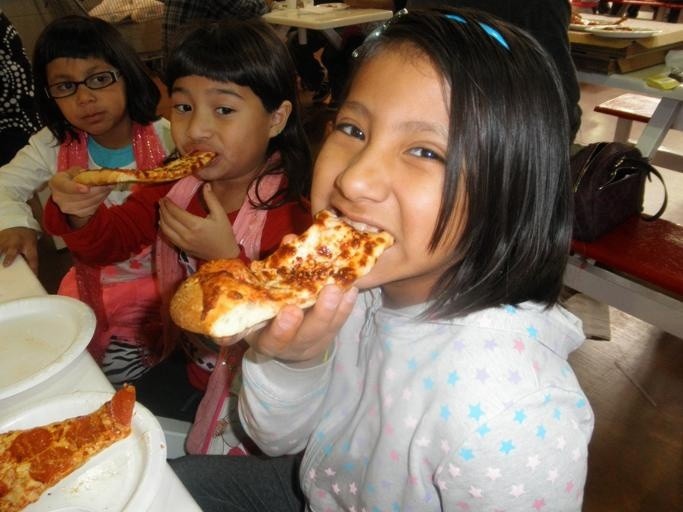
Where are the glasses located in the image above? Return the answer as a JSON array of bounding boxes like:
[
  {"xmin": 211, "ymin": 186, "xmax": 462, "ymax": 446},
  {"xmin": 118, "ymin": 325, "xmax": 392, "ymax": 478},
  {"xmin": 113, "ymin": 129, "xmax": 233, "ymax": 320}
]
[{"xmin": 44, "ymin": 69, "xmax": 122, "ymax": 99}]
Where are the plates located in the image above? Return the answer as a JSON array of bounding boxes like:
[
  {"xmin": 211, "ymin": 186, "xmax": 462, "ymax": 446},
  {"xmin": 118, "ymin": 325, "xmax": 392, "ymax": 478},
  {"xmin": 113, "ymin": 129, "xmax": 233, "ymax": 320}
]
[
  {"xmin": 1, "ymin": 291, "xmax": 96, "ymax": 400},
  {"xmin": 313, "ymin": 2, "xmax": 348, "ymax": 11},
  {"xmin": 2, "ymin": 394, "xmax": 169, "ymax": 512},
  {"xmin": 88, "ymin": 0, "xmax": 167, "ymax": 21},
  {"xmin": 584, "ymin": 24, "xmax": 662, "ymax": 38},
  {"xmin": 569, "ymin": 13, "xmax": 612, "ymax": 32}
]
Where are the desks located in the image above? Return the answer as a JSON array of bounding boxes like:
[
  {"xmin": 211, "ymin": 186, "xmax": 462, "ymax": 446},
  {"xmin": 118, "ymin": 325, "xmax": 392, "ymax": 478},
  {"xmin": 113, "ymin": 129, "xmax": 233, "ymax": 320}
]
[{"xmin": 1, "ymin": 0, "xmax": 683, "ymax": 512}]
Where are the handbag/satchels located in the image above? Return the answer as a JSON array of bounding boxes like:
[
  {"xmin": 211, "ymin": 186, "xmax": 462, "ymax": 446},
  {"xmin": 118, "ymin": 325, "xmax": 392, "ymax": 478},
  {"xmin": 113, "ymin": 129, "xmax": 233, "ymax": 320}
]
[{"xmin": 569, "ymin": 142, "xmax": 649, "ymax": 242}]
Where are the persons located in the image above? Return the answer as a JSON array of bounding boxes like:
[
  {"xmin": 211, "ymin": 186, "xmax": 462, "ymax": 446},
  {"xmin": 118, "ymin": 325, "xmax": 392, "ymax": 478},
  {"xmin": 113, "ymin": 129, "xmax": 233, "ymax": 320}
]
[
  {"xmin": 0, "ymin": 15, "xmax": 185, "ymax": 393},
  {"xmin": 286, "ymin": 1, "xmax": 403, "ymax": 110},
  {"xmin": 36, "ymin": 12, "xmax": 312, "ymax": 426},
  {"xmin": 164, "ymin": 3, "xmax": 598, "ymax": 512},
  {"xmin": 0, "ymin": 16, "xmax": 47, "ymax": 164},
  {"xmin": 161, "ymin": 1, "xmax": 268, "ymax": 69},
  {"xmin": 390, "ymin": 1, "xmax": 585, "ymax": 150}
]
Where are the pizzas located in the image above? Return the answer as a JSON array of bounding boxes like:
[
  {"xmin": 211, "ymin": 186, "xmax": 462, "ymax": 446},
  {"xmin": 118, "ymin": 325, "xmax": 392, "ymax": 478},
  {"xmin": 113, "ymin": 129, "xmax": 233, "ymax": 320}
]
[
  {"xmin": 72, "ymin": 150, "xmax": 217, "ymax": 186},
  {"xmin": 169, "ymin": 209, "xmax": 395, "ymax": 346},
  {"xmin": 0, "ymin": 382, "xmax": 136, "ymax": 512}
]
